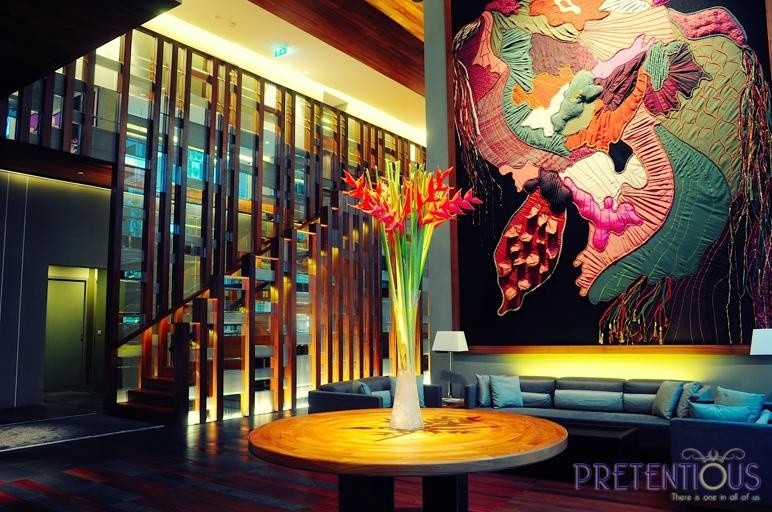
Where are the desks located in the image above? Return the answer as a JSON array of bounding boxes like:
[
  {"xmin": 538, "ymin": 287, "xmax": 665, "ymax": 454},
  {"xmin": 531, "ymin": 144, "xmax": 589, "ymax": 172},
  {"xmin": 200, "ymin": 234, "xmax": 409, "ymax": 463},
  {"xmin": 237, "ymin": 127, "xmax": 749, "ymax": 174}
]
[{"xmin": 247, "ymin": 408, "xmax": 568, "ymax": 512}]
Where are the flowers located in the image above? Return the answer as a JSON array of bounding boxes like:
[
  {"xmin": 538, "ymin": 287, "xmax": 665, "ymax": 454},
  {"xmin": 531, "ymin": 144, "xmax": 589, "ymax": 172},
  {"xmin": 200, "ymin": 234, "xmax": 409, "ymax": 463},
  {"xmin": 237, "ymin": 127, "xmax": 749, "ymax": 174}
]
[{"xmin": 341, "ymin": 159, "xmax": 483, "ymax": 376}]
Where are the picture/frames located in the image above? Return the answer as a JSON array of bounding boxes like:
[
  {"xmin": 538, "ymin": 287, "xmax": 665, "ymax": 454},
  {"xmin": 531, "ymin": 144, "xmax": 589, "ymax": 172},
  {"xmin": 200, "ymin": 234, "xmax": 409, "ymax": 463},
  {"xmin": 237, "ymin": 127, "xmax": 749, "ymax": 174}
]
[{"xmin": 441, "ymin": 0, "xmax": 772, "ymax": 355}]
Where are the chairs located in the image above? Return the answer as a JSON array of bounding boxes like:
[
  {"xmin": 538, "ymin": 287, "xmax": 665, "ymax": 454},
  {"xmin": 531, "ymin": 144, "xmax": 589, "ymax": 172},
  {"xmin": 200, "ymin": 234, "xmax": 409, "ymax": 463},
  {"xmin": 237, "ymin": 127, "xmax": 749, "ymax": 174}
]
[
  {"xmin": 308, "ymin": 376, "xmax": 442, "ymax": 414},
  {"xmin": 669, "ymin": 400, "xmax": 772, "ymax": 508}
]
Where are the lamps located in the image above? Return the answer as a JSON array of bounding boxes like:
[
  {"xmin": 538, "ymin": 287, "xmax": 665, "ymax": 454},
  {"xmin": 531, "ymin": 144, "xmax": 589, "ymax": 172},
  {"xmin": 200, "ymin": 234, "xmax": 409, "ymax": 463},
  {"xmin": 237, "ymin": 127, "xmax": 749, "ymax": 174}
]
[{"xmin": 431, "ymin": 331, "xmax": 469, "ymax": 398}]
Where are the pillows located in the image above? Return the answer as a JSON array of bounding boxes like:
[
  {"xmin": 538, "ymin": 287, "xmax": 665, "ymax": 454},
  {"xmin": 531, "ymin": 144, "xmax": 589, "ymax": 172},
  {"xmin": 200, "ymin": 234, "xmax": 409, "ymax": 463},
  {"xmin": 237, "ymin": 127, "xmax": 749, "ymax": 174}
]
[
  {"xmin": 652, "ymin": 380, "xmax": 700, "ymax": 419},
  {"xmin": 475, "ymin": 374, "xmax": 523, "ymax": 409},
  {"xmin": 687, "ymin": 386, "xmax": 768, "ymax": 422},
  {"xmin": 352, "ymin": 381, "xmax": 371, "ymax": 393}
]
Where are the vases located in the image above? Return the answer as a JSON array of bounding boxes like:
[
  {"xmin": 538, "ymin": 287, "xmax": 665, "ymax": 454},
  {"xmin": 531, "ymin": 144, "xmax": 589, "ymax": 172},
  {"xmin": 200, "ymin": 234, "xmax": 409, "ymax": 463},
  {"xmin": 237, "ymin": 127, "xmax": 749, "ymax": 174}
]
[{"xmin": 388, "ymin": 290, "xmax": 424, "ymax": 431}]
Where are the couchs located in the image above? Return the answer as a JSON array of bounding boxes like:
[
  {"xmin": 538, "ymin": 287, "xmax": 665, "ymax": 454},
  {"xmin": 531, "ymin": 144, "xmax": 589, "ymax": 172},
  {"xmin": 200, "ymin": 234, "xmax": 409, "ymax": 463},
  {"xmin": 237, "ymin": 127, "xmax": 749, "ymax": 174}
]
[{"xmin": 465, "ymin": 376, "xmax": 704, "ymax": 456}]
